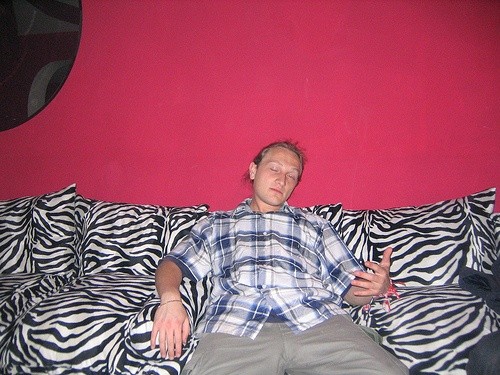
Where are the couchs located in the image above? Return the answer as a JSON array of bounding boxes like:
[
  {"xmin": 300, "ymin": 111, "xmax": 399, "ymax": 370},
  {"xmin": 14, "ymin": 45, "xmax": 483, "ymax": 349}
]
[{"xmin": 0, "ymin": 183, "xmax": 500, "ymax": 374}]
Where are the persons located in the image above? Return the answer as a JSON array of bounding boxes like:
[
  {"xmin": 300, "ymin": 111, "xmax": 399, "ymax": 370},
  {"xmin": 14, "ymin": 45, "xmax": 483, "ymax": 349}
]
[{"xmin": 151, "ymin": 141, "xmax": 410, "ymax": 375}]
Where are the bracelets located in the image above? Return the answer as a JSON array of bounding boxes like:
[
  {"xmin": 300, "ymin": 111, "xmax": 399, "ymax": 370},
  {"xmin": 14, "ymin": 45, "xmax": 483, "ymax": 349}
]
[
  {"xmin": 161, "ymin": 299, "xmax": 182, "ymax": 305},
  {"xmin": 365, "ymin": 279, "xmax": 406, "ymax": 314}
]
